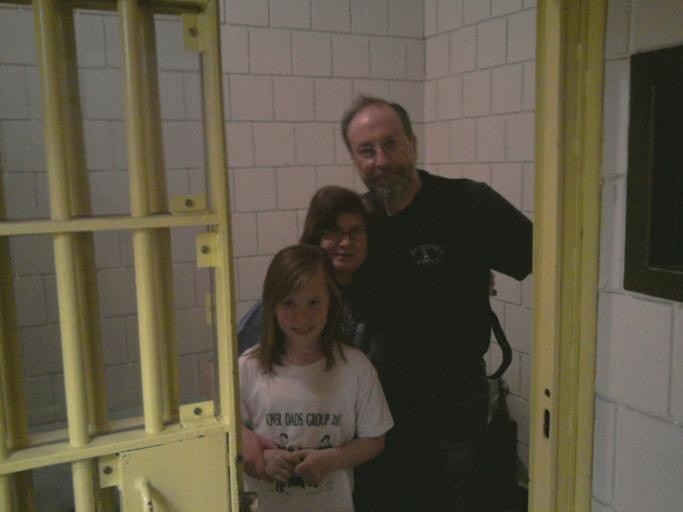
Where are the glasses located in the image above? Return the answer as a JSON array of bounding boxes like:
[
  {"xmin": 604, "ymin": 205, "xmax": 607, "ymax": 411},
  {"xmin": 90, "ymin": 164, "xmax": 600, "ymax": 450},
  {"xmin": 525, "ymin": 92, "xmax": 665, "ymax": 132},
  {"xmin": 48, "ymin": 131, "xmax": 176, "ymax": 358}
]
[
  {"xmin": 352, "ymin": 135, "xmax": 413, "ymax": 160},
  {"xmin": 319, "ymin": 224, "xmax": 369, "ymax": 244}
]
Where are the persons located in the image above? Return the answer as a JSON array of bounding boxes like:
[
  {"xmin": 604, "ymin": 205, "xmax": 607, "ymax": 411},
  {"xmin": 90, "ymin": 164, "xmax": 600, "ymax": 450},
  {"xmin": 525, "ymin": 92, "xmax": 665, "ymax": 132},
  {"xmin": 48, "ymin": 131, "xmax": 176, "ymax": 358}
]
[
  {"xmin": 236, "ymin": 243, "xmax": 396, "ymax": 512},
  {"xmin": 236, "ymin": 185, "xmax": 409, "ymax": 512},
  {"xmin": 341, "ymin": 96, "xmax": 533, "ymax": 512}
]
